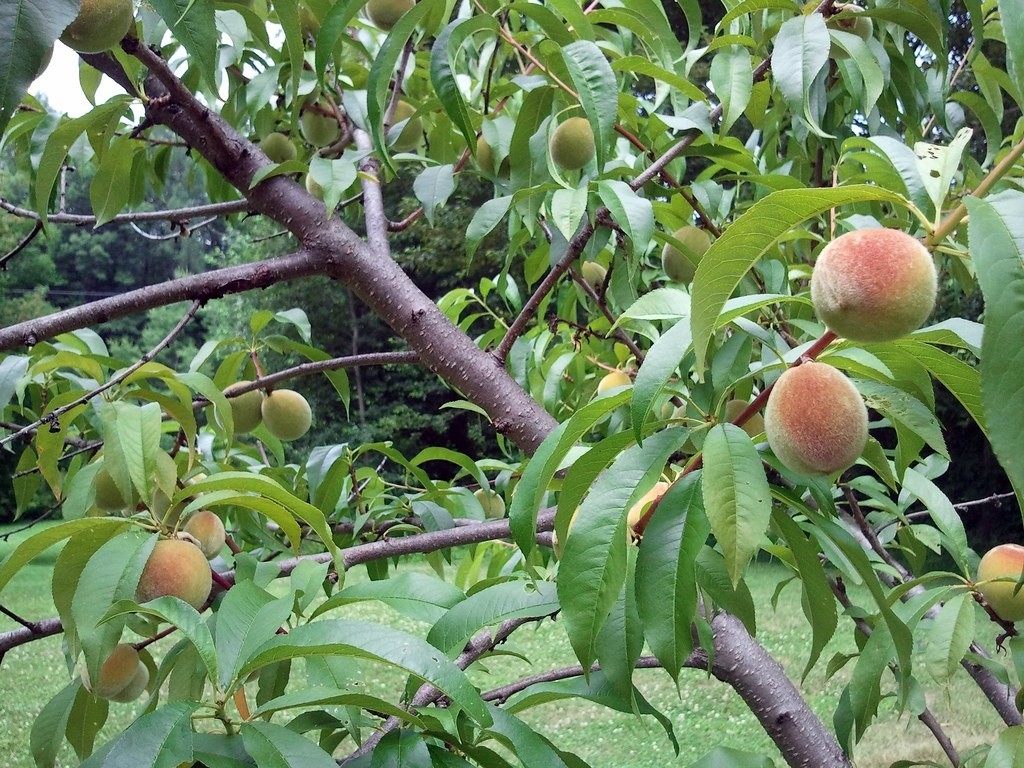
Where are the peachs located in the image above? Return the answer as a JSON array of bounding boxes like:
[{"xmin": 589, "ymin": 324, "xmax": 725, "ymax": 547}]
[{"xmin": 57, "ymin": 2, "xmax": 1024, "ymax": 707}]
